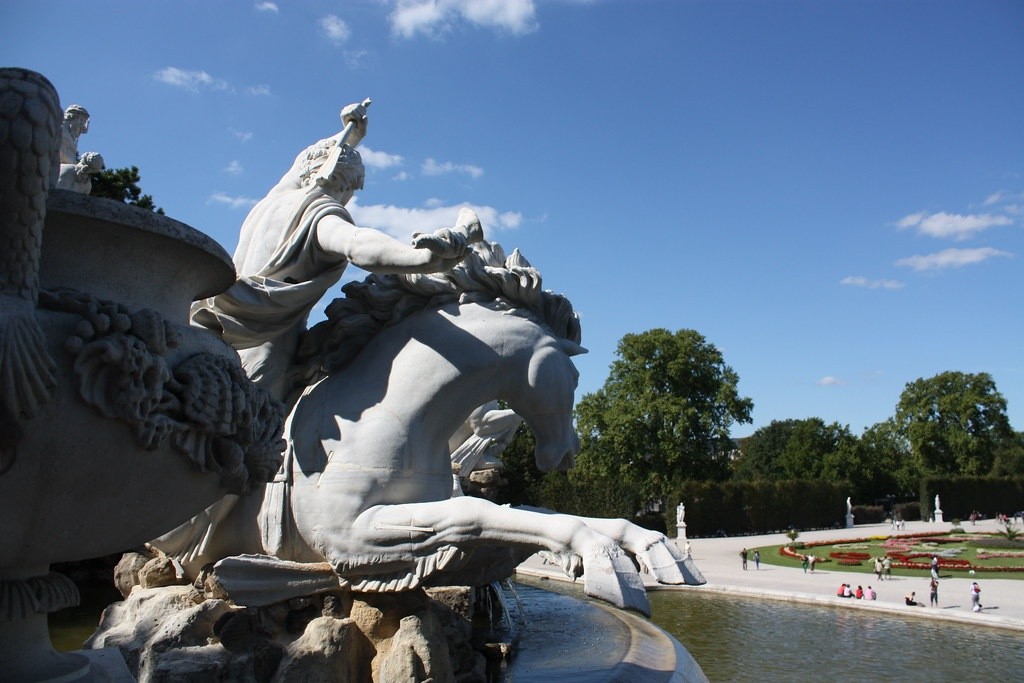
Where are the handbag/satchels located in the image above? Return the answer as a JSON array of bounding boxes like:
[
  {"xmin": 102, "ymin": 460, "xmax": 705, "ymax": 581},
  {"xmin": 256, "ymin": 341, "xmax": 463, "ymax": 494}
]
[{"xmin": 974, "ymin": 584, "xmax": 981, "ymax": 593}]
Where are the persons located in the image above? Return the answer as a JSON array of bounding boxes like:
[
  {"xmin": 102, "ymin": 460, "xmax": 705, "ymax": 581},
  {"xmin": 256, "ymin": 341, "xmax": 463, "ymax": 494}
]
[
  {"xmin": 753, "ymin": 550, "xmax": 759, "ymax": 570},
  {"xmin": 931, "ymin": 555, "xmax": 939, "ymax": 581},
  {"xmin": 55, "ymin": 152, "xmax": 103, "ymax": 195},
  {"xmin": 151, "ymin": 103, "xmax": 470, "ymax": 565},
  {"xmin": 905, "ymin": 591, "xmax": 918, "ymax": 606},
  {"xmin": 935, "ymin": 495, "xmax": 940, "ymax": 510},
  {"xmin": 679, "ymin": 502, "xmax": 685, "ymax": 523},
  {"xmin": 684, "ymin": 541, "xmax": 693, "ymax": 561},
  {"xmin": 838, "ymin": 584, "xmax": 876, "ymax": 601},
  {"xmin": 891, "ymin": 519, "xmax": 905, "ymax": 530},
  {"xmin": 740, "ymin": 548, "xmax": 748, "ymax": 571},
  {"xmin": 847, "ymin": 496, "xmax": 852, "ymax": 516},
  {"xmin": 970, "ymin": 510, "xmax": 1024, "ymax": 526},
  {"xmin": 800, "ymin": 553, "xmax": 816, "ymax": 574},
  {"xmin": 930, "ymin": 579, "xmax": 938, "ymax": 607},
  {"xmin": 61, "ymin": 104, "xmax": 90, "ymax": 164},
  {"xmin": 970, "ymin": 582, "xmax": 982, "ymax": 613},
  {"xmin": 874, "ymin": 556, "xmax": 892, "ymax": 581}
]
